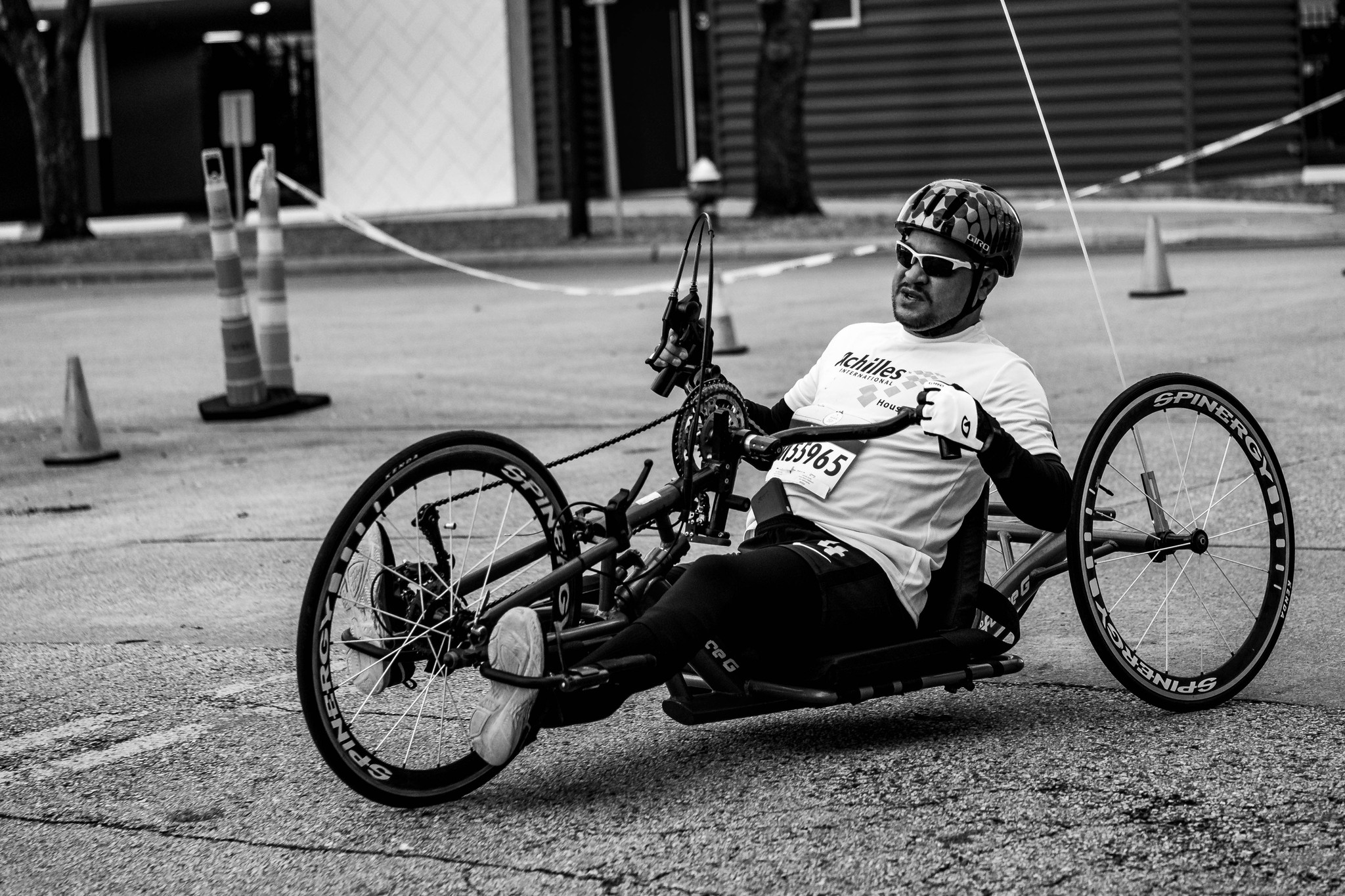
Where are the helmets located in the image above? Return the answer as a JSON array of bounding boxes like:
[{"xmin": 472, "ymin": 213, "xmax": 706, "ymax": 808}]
[{"xmin": 895, "ymin": 178, "xmax": 1023, "ymax": 278}]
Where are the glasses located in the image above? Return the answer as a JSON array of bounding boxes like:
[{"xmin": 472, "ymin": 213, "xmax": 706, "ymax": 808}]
[{"xmin": 895, "ymin": 240, "xmax": 990, "ymax": 277}]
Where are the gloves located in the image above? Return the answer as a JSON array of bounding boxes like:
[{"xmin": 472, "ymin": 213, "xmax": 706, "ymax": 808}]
[{"xmin": 915, "ymin": 381, "xmax": 995, "ymax": 452}]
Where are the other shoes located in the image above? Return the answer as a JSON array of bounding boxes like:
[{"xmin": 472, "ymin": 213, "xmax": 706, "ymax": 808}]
[
  {"xmin": 339, "ymin": 521, "xmax": 419, "ymax": 691},
  {"xmin": 469, "ymin": 606, "xmax": 548, "ymax": 767}
]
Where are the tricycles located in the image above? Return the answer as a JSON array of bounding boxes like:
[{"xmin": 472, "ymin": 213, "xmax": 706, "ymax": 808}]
[{"xmin": 292, "ymin": 210, "xmax": 1299, "ymax": 811}]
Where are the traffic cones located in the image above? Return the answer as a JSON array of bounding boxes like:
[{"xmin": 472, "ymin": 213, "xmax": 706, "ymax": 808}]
[
  {"xmin": 34, "ymin": 352, "xmax": 127, "ymax": 467},
  {"xmin": 1119, "ymin": 212, "xmax": 1188, "ymax": 299},
  {"xmin": 699, "ymin": 263, "xmax": 752, "ymax": 358}
]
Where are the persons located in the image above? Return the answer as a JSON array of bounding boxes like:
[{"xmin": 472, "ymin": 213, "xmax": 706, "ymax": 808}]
[{"xmin": 338, "ymin": 179, "xmax": 1074, "ymax": 766}]
